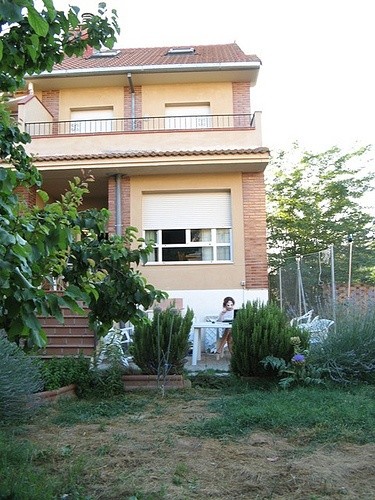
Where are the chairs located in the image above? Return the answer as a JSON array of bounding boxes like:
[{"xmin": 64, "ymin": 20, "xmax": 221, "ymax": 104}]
[
  {"xmin": 216, "ymin": 316, "xmax": 229, "ymax": 358},
  {"xmin": 299, "ymin": 313, "xmax": 319, "ymax": 333},
  {"xmin": 290, "ymin": 309, "xmax": 315, "ymax": 333},
  {"xmin": 302, "ymin": 317, "xmax": 335, "ymax": 356}
]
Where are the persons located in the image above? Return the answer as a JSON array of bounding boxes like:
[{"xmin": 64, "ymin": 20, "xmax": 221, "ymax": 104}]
[{"xmin": 214, "ymin": 297, "xmax": 237, "ymax": 358}]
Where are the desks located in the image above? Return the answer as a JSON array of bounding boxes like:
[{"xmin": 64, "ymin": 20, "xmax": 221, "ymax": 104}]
[{"xmin": 192, "ymin": 320, "xmax": 233, "ymax": 366}]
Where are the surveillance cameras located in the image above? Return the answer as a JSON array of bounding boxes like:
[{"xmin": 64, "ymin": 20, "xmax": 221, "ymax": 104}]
[{"xmin": 348, "ymin": 237, "xmax": 353, "ymax": 242}]
